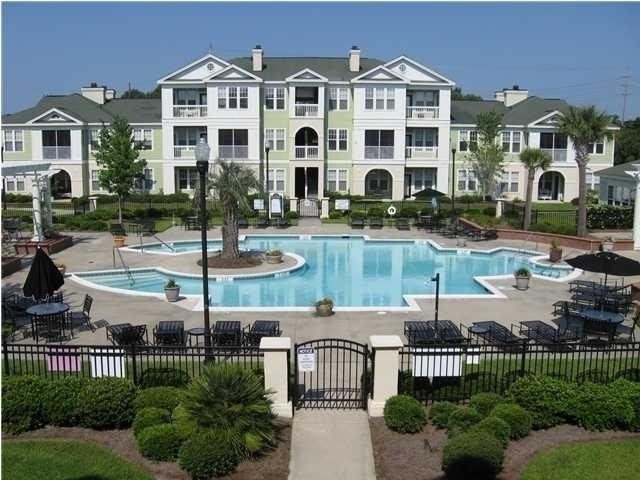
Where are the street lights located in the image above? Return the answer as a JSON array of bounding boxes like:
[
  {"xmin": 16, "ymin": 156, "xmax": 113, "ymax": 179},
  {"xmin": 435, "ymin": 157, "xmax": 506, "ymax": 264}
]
[
  {"xmin": 194, "ymin": 138, "xmax": 216, "ymax": 369},
  {"xmin": 451, "ymin": 143, "xmax": 456, "ymax": 210},
  {"xmin": 264, "ymin": 142, "xmax": 273, "ymax": 194}
]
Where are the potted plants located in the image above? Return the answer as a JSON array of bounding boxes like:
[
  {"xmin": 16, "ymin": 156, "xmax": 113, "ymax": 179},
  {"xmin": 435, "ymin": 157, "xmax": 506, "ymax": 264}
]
[
  {"xmin": 314, "ymin": 298, "xmax": 333, "ymax": 315},
  {"xmin": 600, "ymin": 236, "xmax": 616, "ymax": 253},
  {"xmin": 548, "ymin": 238, "xmax": 563, "ymax": 261},
  {"xmin": 513, "ymin": 268, "xmax": 531, "ymax": 291},
  {"xmin": 112, "ymin": 233, "xmax": 124, "ymax": 246},
  {"xmin": 288, "ymin": 211, "xmax": 299, "ymax": 226},
  {"xmin": 163, "ymin": 279, "xmax": 181, "ymax": 303},
  {"xmin": 55, "ymin": 263, "xmax": 65, "ymax": 275}
]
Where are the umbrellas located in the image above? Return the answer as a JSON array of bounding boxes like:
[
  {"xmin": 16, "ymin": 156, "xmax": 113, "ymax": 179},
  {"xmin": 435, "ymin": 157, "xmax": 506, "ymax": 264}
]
[
  {"xmin": 412, "ymin": 188, "xmax": 445, "ymax": 196},
  {"xmin": 563, "ymin": 252, "xmax": 640, "ymax": 298},
  {"xmin": 22, "ymin": 246, "xmax": 64, "ymax": 303}
]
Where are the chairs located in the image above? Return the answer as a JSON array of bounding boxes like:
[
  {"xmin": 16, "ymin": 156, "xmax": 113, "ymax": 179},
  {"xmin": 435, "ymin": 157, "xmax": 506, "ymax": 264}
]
[
  {"xmin": 104, "ymin": 319, "xmax": 280, "ymax": 355},
  {"xmin": 109, "ymin": 219, "xmax": 156, "ymax": 237},
  {"xmin": 404, "ymin": 278, "xmax": 631, "ymax": 349},
  {"xmin": 238, "ymin": 212, "xmax": 286, "ymax": 228},
  {"xmin": 2, "ymin": 292, "xmax": 94, "ymax": 346},
  {"xmin": 351, "ymin": 212, "xmax": 460, "ymax": 239}
]
